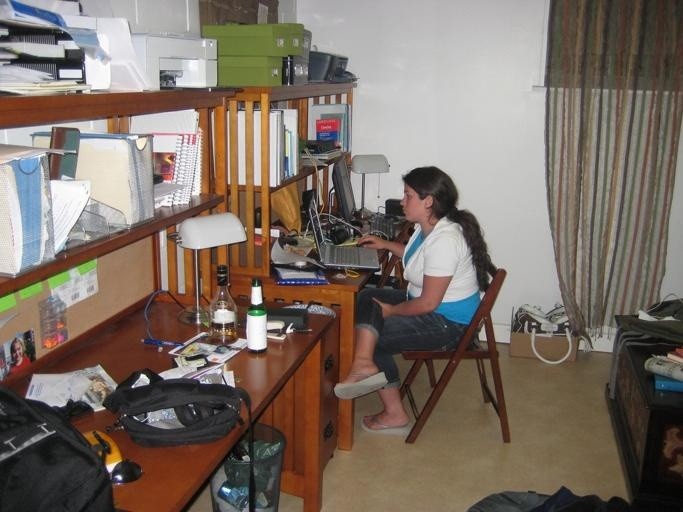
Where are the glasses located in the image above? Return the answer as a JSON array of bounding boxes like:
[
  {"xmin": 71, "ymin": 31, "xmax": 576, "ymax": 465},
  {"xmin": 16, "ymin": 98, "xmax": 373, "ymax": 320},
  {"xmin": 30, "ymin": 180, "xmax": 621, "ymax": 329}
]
[{"xmin": 108, "ymin": 459, "xmax": 144, "ymax": 483}]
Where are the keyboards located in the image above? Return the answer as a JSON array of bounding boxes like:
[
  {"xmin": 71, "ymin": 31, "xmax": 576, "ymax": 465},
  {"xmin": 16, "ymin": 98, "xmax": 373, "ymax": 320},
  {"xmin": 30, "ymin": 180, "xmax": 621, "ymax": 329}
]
[{"xmin": 368, "ymin": 212, "xmax": 395, "ymax": 241}]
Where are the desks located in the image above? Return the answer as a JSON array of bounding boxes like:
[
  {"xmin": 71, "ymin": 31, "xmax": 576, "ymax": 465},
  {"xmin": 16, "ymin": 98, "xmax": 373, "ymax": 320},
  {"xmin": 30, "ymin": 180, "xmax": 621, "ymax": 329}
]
[
  {"xmin": 4, "ymin": 299, "xmax": 335, "ymax": 512},
  {"xmin": 197, "ymin": 210, "xmax": 413, "ymax": 452}
]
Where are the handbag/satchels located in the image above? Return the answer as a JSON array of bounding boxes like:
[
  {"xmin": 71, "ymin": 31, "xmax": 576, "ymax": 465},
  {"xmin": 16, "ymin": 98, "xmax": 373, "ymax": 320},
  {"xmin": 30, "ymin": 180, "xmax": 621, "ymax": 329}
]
[
  {"xmin": 513, "ymin": 303, "xmax": 575, "ymax": 337},
  {"xmin": 0, "ymin": 382, "xmax": 114, "ymax": 512},
  {"xmin": 102, "ymin": 367, "xmax": 246, "ymax": 447}
]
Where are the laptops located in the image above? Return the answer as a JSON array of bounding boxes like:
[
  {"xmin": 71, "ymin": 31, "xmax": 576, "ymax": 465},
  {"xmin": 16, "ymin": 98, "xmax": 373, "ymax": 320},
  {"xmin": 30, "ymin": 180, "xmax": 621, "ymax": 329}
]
[{"xmin": 308, "ymin": 195, "xmax": 380, "ymax": 271}]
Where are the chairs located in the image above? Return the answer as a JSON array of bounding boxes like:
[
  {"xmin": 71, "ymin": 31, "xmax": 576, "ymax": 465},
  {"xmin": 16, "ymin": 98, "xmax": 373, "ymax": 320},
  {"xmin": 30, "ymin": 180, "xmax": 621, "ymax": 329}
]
[{"xmin": 398, "ymin": 262, "xmax": 512, "ymax": 444}]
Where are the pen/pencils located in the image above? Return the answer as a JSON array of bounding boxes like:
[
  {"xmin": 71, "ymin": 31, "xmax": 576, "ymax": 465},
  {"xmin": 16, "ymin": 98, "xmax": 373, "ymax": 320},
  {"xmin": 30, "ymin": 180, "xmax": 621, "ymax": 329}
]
[
  {"xmin": 141, "ymin": 339, "xmax": 184, "ymax": 346},
  {"xmin": 294, "ymin": 328, "xmax": 312, "ymax": 333}
]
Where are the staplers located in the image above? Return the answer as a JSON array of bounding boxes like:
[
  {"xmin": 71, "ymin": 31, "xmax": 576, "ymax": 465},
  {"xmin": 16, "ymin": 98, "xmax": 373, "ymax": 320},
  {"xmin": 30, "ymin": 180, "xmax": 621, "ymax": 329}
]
[{"xmin": 267, "ymin": 321, "xmax": 287, "ymax": 341}]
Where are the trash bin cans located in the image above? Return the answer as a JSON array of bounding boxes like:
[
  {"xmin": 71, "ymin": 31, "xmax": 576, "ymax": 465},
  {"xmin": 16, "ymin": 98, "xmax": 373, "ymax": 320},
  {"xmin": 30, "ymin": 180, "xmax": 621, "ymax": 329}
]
[{"xmin": 208, "ymin": 423, "xmax": 286, "ymax": 512}]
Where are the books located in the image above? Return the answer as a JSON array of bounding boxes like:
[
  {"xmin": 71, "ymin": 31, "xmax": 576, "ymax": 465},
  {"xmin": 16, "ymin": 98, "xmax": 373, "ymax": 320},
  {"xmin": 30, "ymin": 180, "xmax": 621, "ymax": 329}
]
[
  {"xmin": 211, "ymin": 103, "xmax": 353, "ymax": 188},
  {"xmin": 274, "ymin": 265, "xmax": 331, "ymax": 285},
  {"xmin": 644, "ymin": 348, "xmax": 683, "ymax": 393},
  {"xmin": 128, "ymin": 108, "xmax": 203, "ymax": 209}
]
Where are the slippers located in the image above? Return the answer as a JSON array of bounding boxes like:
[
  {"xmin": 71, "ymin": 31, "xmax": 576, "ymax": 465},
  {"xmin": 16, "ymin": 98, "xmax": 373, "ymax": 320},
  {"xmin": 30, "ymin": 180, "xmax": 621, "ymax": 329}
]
[
  {"xmin": 362, "ymin": 414, "xmax": 411, "ymax": 436},
  {"xmin": 334, "ymin": 371, "xmax": 389, "ymax": 400}
]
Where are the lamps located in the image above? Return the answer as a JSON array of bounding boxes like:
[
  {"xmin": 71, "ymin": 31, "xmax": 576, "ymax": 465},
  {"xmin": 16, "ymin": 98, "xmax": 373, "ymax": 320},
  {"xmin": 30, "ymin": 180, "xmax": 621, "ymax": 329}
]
[
  {"xmin": 167, "ymin": 211, "xmax": 247, "ymax": 327},
  {"xmin": 349, "ymin": 153, "xmax": 391, "ymax": 220}
]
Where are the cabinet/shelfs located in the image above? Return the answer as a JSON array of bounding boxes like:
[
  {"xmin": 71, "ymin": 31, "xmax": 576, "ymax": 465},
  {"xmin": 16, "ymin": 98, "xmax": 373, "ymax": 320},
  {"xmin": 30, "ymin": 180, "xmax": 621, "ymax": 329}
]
[
  {"xmin": 604, "ymin": 313, "xmax": 683, "ymax": 511},
  {"xmin": 197, "ymin": 79, "xmax": 358, "ymax": 279},
  {"xmin": 0, "ymin": 86, "xmax": 225, "ymax": 386}
]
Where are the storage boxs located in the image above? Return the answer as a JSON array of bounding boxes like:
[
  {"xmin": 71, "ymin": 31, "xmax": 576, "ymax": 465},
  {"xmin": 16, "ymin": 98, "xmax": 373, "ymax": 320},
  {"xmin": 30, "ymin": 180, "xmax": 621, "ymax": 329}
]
[
  {"xmin": 510, "ymin": 328, "xmax": 594, "ymax": 363},
  {"xmin": 201, "ymin": 22, "xmax": 304, "ymax": 88}
]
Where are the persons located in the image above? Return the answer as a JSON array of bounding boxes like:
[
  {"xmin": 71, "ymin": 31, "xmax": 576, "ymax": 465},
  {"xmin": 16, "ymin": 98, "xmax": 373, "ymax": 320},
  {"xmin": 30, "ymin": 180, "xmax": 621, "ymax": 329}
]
[
  {"xmin": 8, "ymin": 338, "xmax": 31, "ymax": 374},
  {"xmin": 333, "ymin": 166, "xmax": 500, "ymax": 435}
]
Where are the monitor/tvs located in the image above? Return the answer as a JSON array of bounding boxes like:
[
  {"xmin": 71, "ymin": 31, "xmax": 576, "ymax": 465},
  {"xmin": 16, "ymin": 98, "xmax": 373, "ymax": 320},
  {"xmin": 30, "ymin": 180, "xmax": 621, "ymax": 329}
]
[{"xmin": 331, "ymin": 152, "xmax": 364, "ymax": 230}]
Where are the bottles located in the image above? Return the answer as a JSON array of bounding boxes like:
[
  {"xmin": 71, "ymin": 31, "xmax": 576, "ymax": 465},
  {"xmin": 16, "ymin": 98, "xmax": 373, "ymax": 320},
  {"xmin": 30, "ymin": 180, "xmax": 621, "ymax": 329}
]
[
  {"xmin": 209, "ymin": 264, "xmax": 238, "ymax": 344},
  {"xmin": 245, "ymin": 277, "xmax": 268, "ymax": 354}
]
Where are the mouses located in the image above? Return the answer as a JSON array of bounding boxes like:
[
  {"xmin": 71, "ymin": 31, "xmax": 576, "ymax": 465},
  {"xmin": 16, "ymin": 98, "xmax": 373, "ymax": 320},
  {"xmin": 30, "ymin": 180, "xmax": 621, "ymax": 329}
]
[
  {"xmin": 385, "ymin": 215, "xmax": 400, "ymax": 222},
  {"xmin": 356, "ymin": 241, "xmax": 372, "ymax": 247}
]
